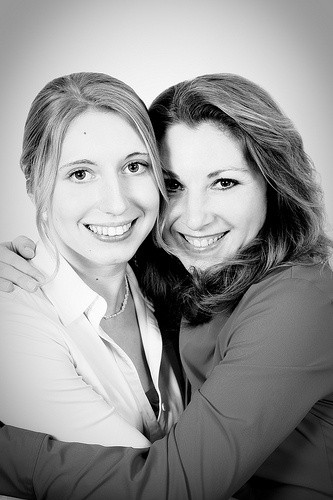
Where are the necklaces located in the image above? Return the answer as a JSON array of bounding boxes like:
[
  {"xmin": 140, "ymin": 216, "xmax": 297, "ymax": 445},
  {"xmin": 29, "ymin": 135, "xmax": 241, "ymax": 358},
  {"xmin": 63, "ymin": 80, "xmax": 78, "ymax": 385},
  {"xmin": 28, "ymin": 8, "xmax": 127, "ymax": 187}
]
[{"xmin": 101, "ymin": 273, "xmax": 129, "ymax": 320}]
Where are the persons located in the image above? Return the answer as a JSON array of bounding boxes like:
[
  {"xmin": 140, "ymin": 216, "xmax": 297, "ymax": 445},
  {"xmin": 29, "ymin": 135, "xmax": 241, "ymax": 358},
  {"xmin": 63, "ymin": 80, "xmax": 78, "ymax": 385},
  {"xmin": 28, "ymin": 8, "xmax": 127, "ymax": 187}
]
[
  {"xmin": 0, "ymin": 71, "xmax": 185, "ymax": 500},
  {"xmin": 0, "ymin": 72, "xmax": 333, "ymax": 500}
]
